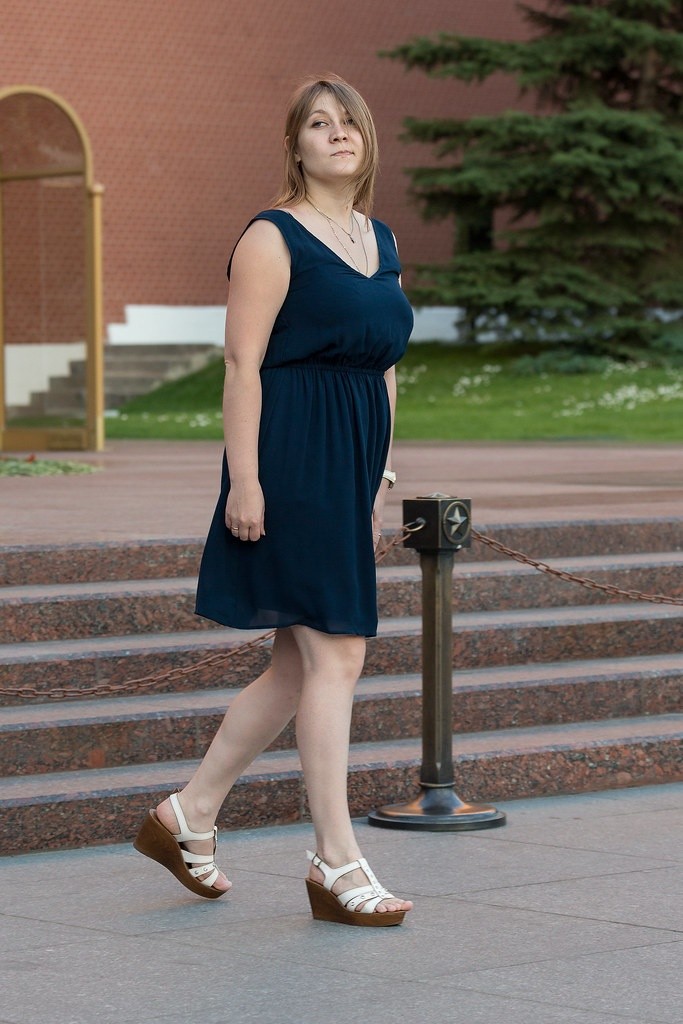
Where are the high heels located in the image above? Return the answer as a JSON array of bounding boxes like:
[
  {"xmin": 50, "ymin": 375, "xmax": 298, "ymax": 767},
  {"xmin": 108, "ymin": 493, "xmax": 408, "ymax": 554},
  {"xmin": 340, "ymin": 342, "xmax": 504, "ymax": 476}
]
[
  {"xmin": 303, "ymin": 850, "xmax": 406, "ymax": 927},
  {"xmin": 131, "ymin": 793, "xmax": 227, "ymax": 899}
]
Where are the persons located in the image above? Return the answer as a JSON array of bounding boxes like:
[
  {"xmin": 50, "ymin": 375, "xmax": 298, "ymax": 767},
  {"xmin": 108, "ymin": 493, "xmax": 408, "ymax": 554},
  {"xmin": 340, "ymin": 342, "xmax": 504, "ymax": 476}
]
[{"xmin": 127, "ymin": 72, "xmax": 417, "ymax": 926}]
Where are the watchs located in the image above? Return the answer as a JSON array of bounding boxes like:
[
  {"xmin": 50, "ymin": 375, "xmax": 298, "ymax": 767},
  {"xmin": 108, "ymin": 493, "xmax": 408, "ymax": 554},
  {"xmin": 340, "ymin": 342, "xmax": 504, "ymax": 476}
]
[{"xmin": 382, "ymin": 468, "xmax": 398, "ymax": 490}]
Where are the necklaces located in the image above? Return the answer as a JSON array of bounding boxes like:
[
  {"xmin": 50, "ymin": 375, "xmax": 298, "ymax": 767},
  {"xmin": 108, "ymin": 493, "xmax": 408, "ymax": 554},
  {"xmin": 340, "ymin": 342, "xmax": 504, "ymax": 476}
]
[{"xmin": 301, "ymin": 191, "xmax": 372, "ymax": 279}]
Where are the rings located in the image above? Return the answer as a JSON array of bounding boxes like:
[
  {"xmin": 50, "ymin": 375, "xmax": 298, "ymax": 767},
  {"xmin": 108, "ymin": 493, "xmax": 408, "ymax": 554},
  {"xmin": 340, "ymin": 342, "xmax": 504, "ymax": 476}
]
[{"xmin": 230, "ymin": 525, "xmax": 239, "ymax": 531}]
